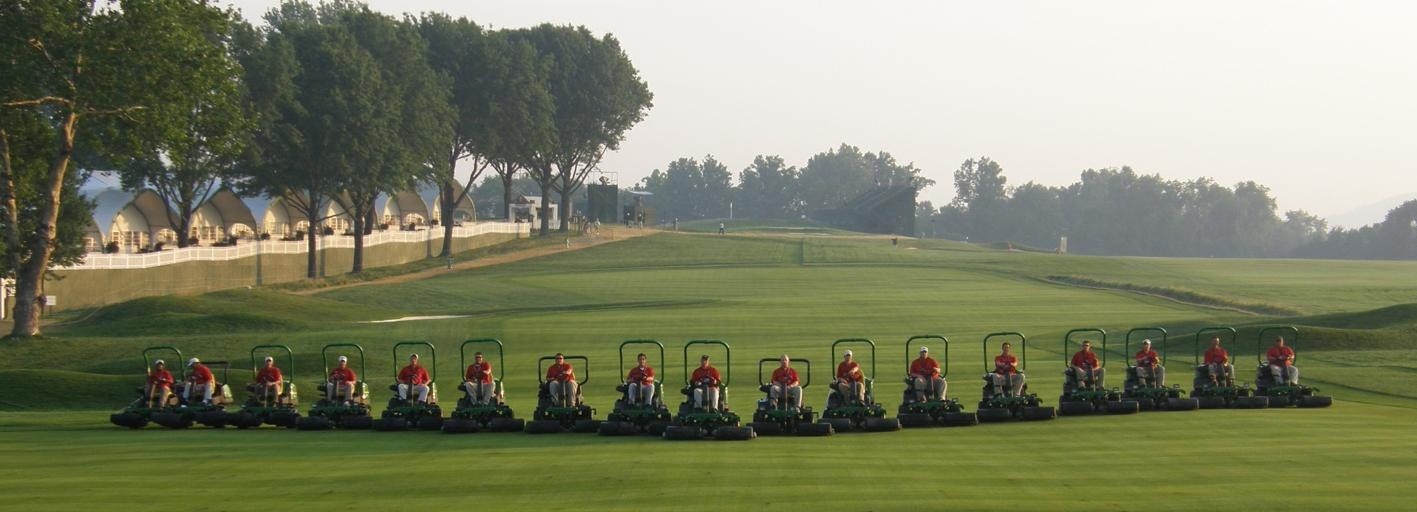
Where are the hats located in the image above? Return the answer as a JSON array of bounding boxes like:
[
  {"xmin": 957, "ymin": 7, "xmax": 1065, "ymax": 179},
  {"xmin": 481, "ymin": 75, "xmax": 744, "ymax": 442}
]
[
  {"xmin": 188, "ymin": 358, "xmax": 200, "ymax": 367},
  {"xmin": 920, "ymin": 346, "xmax": 928, "ymax": 352},
  {"xmin": 155, "ymin": 360, "xmax": 164, "ymax": 365},
  {"xmin": 1143, "ymin": 339, "xmax": 1151, "ymax": 344},
  {"xmin": 475, "ymin": 352, "xmax": 481, "ymax": 357},
  {"xmin": 844, "ymin": 350, "xmax": 852, "ymax": 357},
  {"xmin": 339, "ymin": 356, "xmax": 347, "ymax": 362},
  {"xmin": 410, "ymin": 353, "xmax": 418, "ymax": 359},
  {"xmin": 265, "ymin": 357, "xmax": 273, "ymax": 362}
]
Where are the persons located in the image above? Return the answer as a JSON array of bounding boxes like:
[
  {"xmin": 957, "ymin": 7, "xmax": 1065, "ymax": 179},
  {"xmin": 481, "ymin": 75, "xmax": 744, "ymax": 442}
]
[
  {"xmin": 463, "ymin": 351, "xmax": 497, "ymax": 409},
  {"xmin": 624, "ymin": 351, "xmax": 656, "ymax": 411},
  {"xmin": 1202, "ymin": 334, "xmax": 1237, "ymax": 389},
  {"xmin": 830, "ymin": 349, "xmax": 866, "ymax": 408},
  {"xmin": 989, "ymin": 342, "xmax": 1025, "ymax": 399},
  {"xmin": 689, "ymin": 352, "xmax": 721, "ymax": 414},
  {"xmin": 323, "ymin": 354, "xmax": 357, "ymax": 407},
  {"xmin": 178, "ymin": 357, "xmax": 217, "ymax": 408},
  {"xmin": 142, "ymin": 359, "xmax": 175, "ymax": 411},
  {"xmin": 545, "ymin": 352, "xmax": 578, "ymax": 409},
  {"xmin": 909, "ymin": 345, "xmax": 947, "ymax": 404},
  {"xmin": 717, "ymin": 220, "xmax": 724, "ymax": 236},
  {"xmin": 765, "ymin": 351, "xmax": 803, "ymax": 414},
  {"xmin": 1134, "ymin": 337, "xmax": 1169, "ymax": 391},
  {"xmin": 671, "ymin": 217, "xmax": 680, "ymax": 232},
  {"xmin": 395, "ymin": 352, "xmax": 433, "ymax": 408},
  {"xmin": 1264, "ymin": 335, "xmax": 1298, "ymax": 387},
  {"xmin": 575, "ymin": 208, "xmax": 644, "ymax": 242},
  {"xmin": 249, "ymin": 355, "xmax": 282, "ymax": 410},
  {"xmin": 1068, "ymin": 339, "xmax": 1106, "ymax": 392}
]
